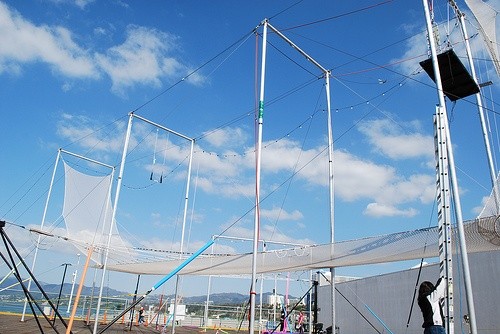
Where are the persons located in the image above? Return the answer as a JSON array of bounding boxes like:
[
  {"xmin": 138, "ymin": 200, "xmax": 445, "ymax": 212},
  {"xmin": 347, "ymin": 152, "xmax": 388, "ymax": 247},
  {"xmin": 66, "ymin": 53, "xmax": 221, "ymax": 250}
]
[
  {"xmin": 137, "ymin": 306, "xmax": 148, "ymax": 326},
  {"xmin": 415, "ymin": 272, "xmax": 451, "ymax": 334},
  {"xmin": 277, "ymin": 304, "xmax": 306, "ymax": 333}
]
